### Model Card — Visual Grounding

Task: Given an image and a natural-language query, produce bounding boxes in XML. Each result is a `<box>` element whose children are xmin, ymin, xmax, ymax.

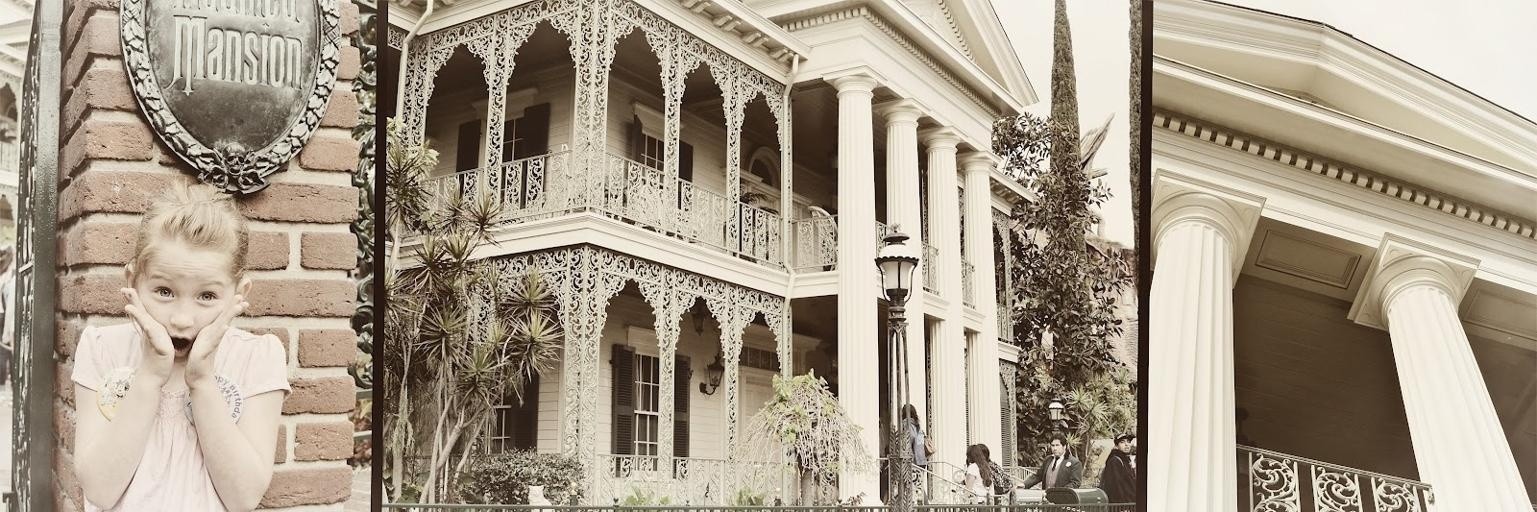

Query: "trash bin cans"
<box><xmin>1011</xmin><ymin>489</ymin><xmax>1110</xmax><ymax>512</ymax></box>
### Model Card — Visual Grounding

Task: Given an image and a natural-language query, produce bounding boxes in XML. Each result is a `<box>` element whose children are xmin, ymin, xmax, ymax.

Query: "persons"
<box><xmin>70</xmin><ymin>179</ymin><xmax>296</xmax><ymax>512</ymax></box>
<box><xmin>1095</xmin><ymin>432</ymin><xmax>1136</xmax><ymax>511</ymax></box>
<box><xmin>1017</xmin><ymin>433</ymin><xmax>1083</xmax><ymax>490</ymax></box>
<box><xmin>887</xmin><ymin>403</ymin><xmax>930</xmax><ymax>504</ymax></box>
<box><xmin>960</xmin><ymin>444</ymin><xmax>996</xmax><ymax>512</ymax></box>
<box><xmin>975</xmin><ymin>442</ymin><xmax>1003</xmax><ymax>511</ymax></box>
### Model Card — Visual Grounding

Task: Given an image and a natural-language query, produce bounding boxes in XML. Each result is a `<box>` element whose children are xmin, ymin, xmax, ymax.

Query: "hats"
<box><xmin>1114</xmin><ymin>433</ymin><xmax>1134</xmax><ymax>446</ymax></box>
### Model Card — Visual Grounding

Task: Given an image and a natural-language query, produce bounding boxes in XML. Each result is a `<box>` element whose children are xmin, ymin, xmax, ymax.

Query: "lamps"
<box><xmin>692</xmin><ymin>303</ymin><xmax>708</xmax><ymax>335</ymax></box>
<box><xmin>699</xmin><ymin>353</ymin><xmax>725</xmax><ymax>396</ymax></box>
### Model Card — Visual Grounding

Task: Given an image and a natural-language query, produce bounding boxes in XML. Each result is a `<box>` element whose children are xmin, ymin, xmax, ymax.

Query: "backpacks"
<box><xmin>987</xmin><ymin>460</ymin><xmax>1015</xmax><ymax>494</ymax></box>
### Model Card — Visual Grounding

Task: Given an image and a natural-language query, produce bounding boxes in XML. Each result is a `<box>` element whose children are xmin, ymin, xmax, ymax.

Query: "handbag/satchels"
<box><xmin>923</xmin><ymin>431</ymin><xmax>937</xmax><ymax>457</ymax></box>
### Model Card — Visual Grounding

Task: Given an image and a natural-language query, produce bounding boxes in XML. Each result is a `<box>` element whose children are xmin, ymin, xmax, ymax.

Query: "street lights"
<box><xmin>1048</xmin><ymin>395</ymin><xmax>1064</xmax><ymax>440</ymax></box>
<box><xmin>873</xmin><ymin>225</ymin><xmax>920</xmax><ymax>512</ymax></box>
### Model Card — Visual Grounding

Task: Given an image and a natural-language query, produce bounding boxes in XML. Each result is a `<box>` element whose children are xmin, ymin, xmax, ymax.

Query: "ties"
<box><xmin>1052</xmin><ymin>456</ymin><xmax>1060</xmax><ymax>472</ymax></box>
